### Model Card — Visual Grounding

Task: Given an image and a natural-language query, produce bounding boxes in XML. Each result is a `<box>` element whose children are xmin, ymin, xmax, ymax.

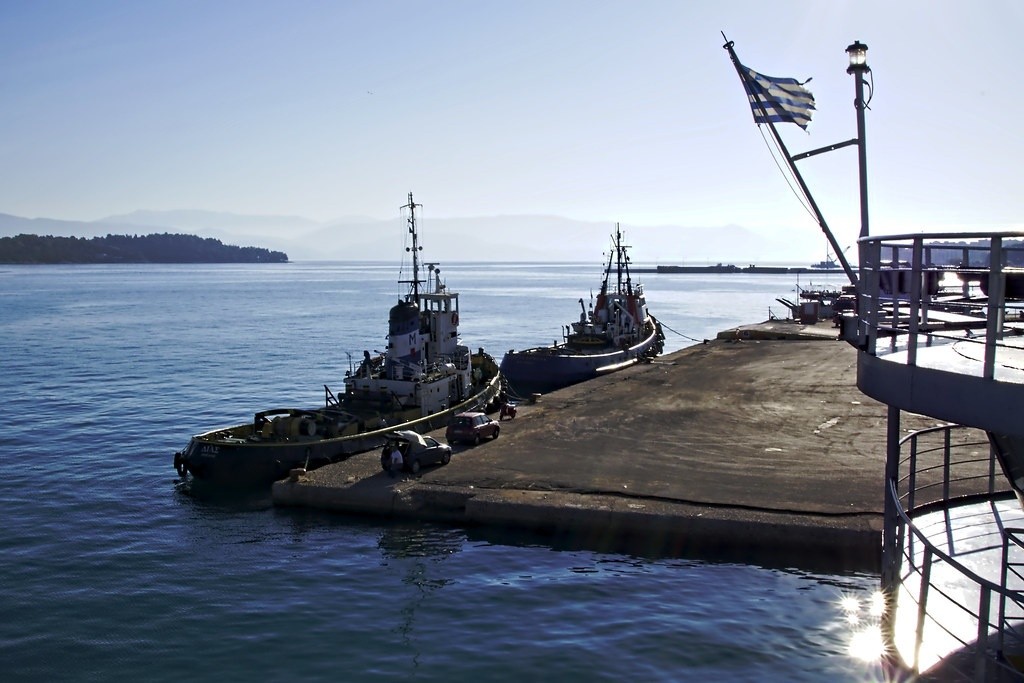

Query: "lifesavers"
<box><xmin>173</xmin><ymin>451</ymin><xmax>188</xmax><ymax>479</ymax></box>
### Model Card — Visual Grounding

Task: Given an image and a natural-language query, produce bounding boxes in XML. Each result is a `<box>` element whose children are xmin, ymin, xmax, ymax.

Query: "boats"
<box><xmin>173</xmin><ymin>188</ymin><xmax>511</xmax><ymax>502</ymax></box>
<box><xmin>499</xmin><ymin>221</ymin><xmax>667</xmax><ymax>404</ymax></box>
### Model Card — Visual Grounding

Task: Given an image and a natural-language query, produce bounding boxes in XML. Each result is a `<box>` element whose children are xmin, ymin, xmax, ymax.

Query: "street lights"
<box><xmin>844</xmin><ymin>38</ymin><xmax>871</xmax><ymax>237</ymax></box>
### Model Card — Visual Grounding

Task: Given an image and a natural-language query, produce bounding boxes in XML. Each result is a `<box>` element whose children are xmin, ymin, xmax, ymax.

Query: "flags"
<box><xmin>730</xmin><ymin>55</ymin><xmax>818</xmax><ymax>136</ymax></box>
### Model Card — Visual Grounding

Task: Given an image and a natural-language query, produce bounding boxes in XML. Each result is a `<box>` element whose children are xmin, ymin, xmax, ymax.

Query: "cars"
<box><xmin>446</xmin><ymin>411</ymin><xmax>501</xmax><ymax>447</ymax></box>
<box><xmin>380</xmin><ymin>430</ymin><xmax>453</xmax><ymax>475</ymax></box>
